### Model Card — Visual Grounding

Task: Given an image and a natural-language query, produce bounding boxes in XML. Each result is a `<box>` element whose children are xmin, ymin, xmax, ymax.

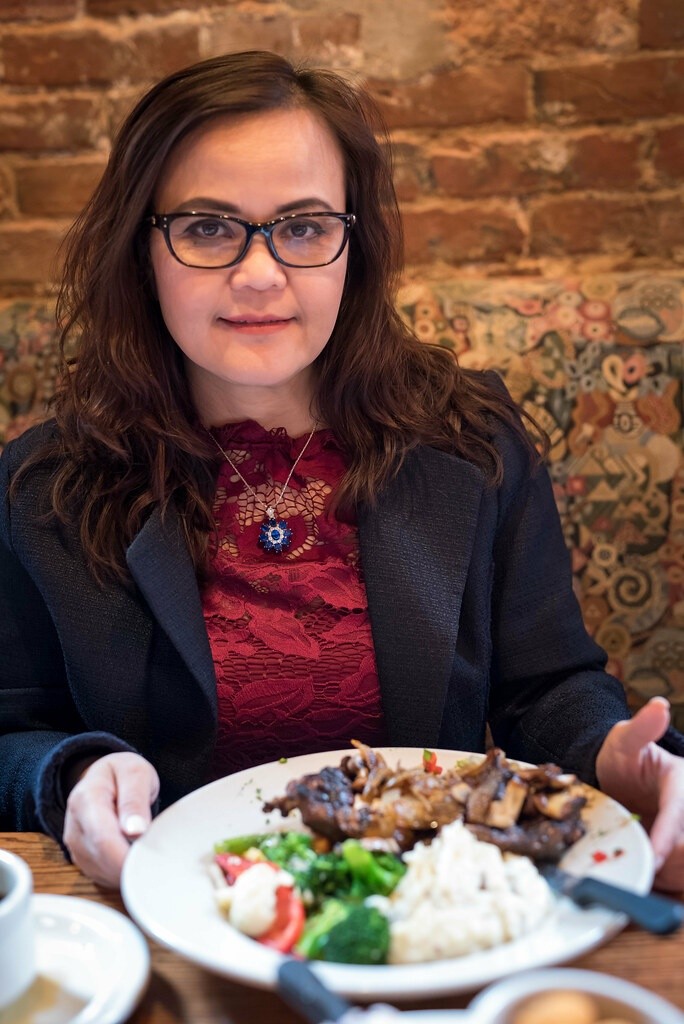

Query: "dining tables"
<box><xmin>0</xmin><ymin>829</ymin><xmax>684</xmax><ymax>1024</ymax></box>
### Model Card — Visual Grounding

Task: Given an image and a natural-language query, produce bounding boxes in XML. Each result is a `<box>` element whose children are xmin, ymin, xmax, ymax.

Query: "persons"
<box><xmin>0</xmin><ymin>48</ymin><xmax>684</xmax><ymax>894</ymax></box>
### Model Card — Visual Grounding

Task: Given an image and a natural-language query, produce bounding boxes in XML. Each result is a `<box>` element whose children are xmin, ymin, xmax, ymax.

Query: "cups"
<box><xmin>368</xmin><ymin>966</ymin><xmax>684</xmax><ymax>1024</ymax></box>
<box><xmin>0</xmin><ymin>847</ymin><xmax>39</xmax><ymax>1002</ymax></box>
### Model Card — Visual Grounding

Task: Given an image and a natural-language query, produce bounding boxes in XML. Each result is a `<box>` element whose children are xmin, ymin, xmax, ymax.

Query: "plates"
<box><xmin>0</xmin><ymin>893</ymin><xmax>150</xmax><ymax>1024</ymax></box>
<box><xmin>121</xmin><ymin>746</ymin><xmax>656</xmax><ymax>999</ymax></box>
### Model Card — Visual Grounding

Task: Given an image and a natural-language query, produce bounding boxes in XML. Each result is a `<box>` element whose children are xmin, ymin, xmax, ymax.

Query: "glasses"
<box><xmin>146</xmin><ymin>212</ymin><xmax>357</xmax><ymax>269</ymax></box>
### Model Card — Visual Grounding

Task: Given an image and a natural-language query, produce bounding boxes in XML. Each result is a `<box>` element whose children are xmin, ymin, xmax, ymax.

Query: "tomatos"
<box><xmin>217</xmin><ymin>849</ymin><xmax>303</xmax><ymax>950</ymax></box>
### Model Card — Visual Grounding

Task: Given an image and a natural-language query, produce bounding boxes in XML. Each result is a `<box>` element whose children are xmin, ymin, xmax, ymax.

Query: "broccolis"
<box><xmin>211</xmin><ymin>829</ymin><xmax>406</xmax><ymax>965</ymax></box>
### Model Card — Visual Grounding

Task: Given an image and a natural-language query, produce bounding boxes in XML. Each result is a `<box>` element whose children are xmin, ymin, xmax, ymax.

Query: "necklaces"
<box><xmin>201</xmin><ymin>415</ymin><xmax>322</xmax><ymax>555</ymax></box>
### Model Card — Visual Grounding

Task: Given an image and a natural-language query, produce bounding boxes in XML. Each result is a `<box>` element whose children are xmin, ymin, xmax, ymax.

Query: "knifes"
<box><xmin>542</xmin><ymin>864</ymin><xmax>684</xmax><ymax>936</ymax></box>
